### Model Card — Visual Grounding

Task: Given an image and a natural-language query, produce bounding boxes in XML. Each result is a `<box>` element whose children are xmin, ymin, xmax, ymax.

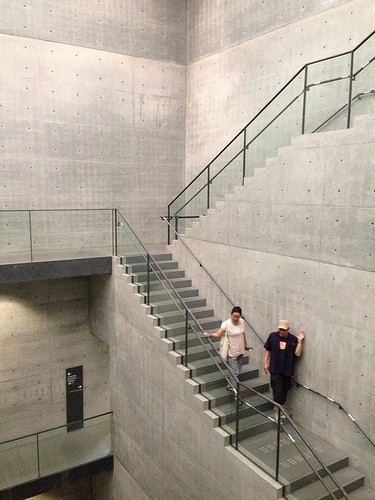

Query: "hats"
<box><xmin>277</xmin><ymin>320</ymin><xmax>290</xmax><ymax>330</ymax></box>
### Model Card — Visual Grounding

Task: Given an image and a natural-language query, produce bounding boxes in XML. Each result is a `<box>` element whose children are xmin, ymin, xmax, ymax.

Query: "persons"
<box><xmin>204</xmin><ymin>307</ymin><xmax>250</xmax><ymax>405</ymax></box>
<box><xmin>263</xmin><ymin>319</ymin><xmax>306</xmax><ymax>415</ymax></box>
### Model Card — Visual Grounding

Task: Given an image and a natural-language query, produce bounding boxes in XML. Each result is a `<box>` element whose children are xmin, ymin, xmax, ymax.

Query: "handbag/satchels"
<box><xmin>219</xmin><ymin>321</ymin><xmax>229</xmax><ymax>363</ymax></box>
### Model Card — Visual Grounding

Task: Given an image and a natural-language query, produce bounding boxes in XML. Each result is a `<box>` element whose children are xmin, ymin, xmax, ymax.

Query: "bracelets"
<box><xmin>298</xmin><ymin>341</ymin><xmax>302</xmax><ymax>343</ymax></box>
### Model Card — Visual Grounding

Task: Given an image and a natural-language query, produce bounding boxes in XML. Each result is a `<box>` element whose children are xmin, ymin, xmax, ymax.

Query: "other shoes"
<box><xmin>226</xmin><ymin>384</ymin><xmax>234</xmax><ymax>392</ymax></box>
<box><xmin>234</xmin><ymin>395</ymin><xmax>244</xmax><ymax>407</ymax></box>
<box><xmin>274</xmin><ymin>407</ymin><xmax>288</xmax><ymax>421</ymax></box>
